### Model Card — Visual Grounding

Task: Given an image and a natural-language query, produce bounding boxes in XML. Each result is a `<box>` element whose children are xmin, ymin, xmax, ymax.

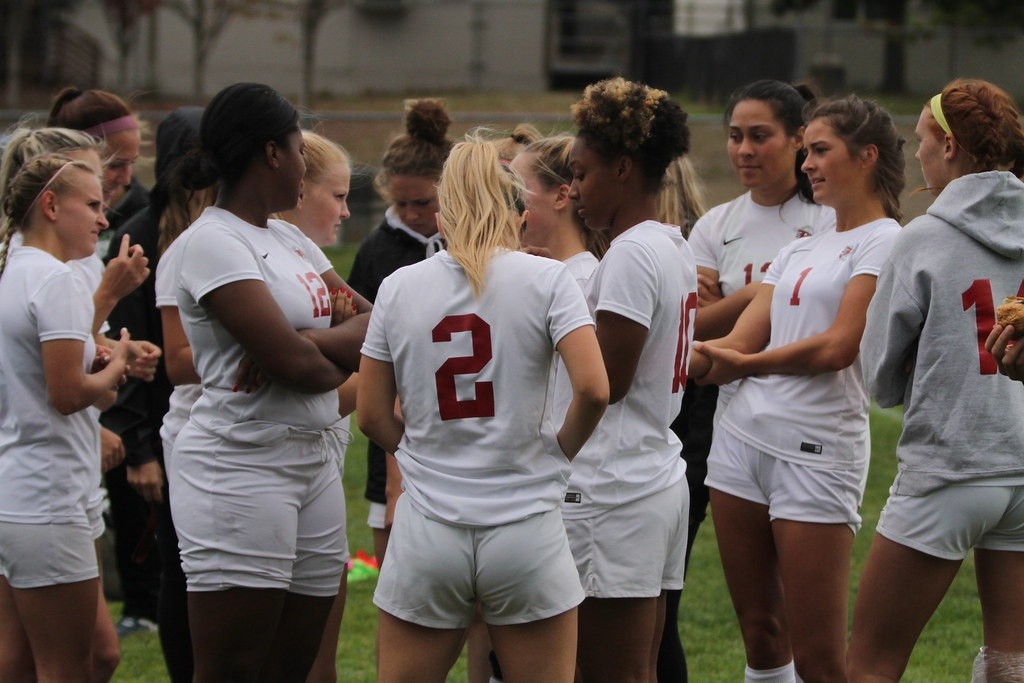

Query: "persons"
<box><xmin>0</xmin><ymin>76</ymin><xmax>1024</xmax><ymax>683</ymax></box>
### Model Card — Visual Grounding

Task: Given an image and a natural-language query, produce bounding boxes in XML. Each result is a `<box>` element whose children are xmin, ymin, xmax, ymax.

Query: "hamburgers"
<box><xmin>995</xmin><ymin>295</ymin><xmax>1024</xmax><ymax>338</ymax></box>
